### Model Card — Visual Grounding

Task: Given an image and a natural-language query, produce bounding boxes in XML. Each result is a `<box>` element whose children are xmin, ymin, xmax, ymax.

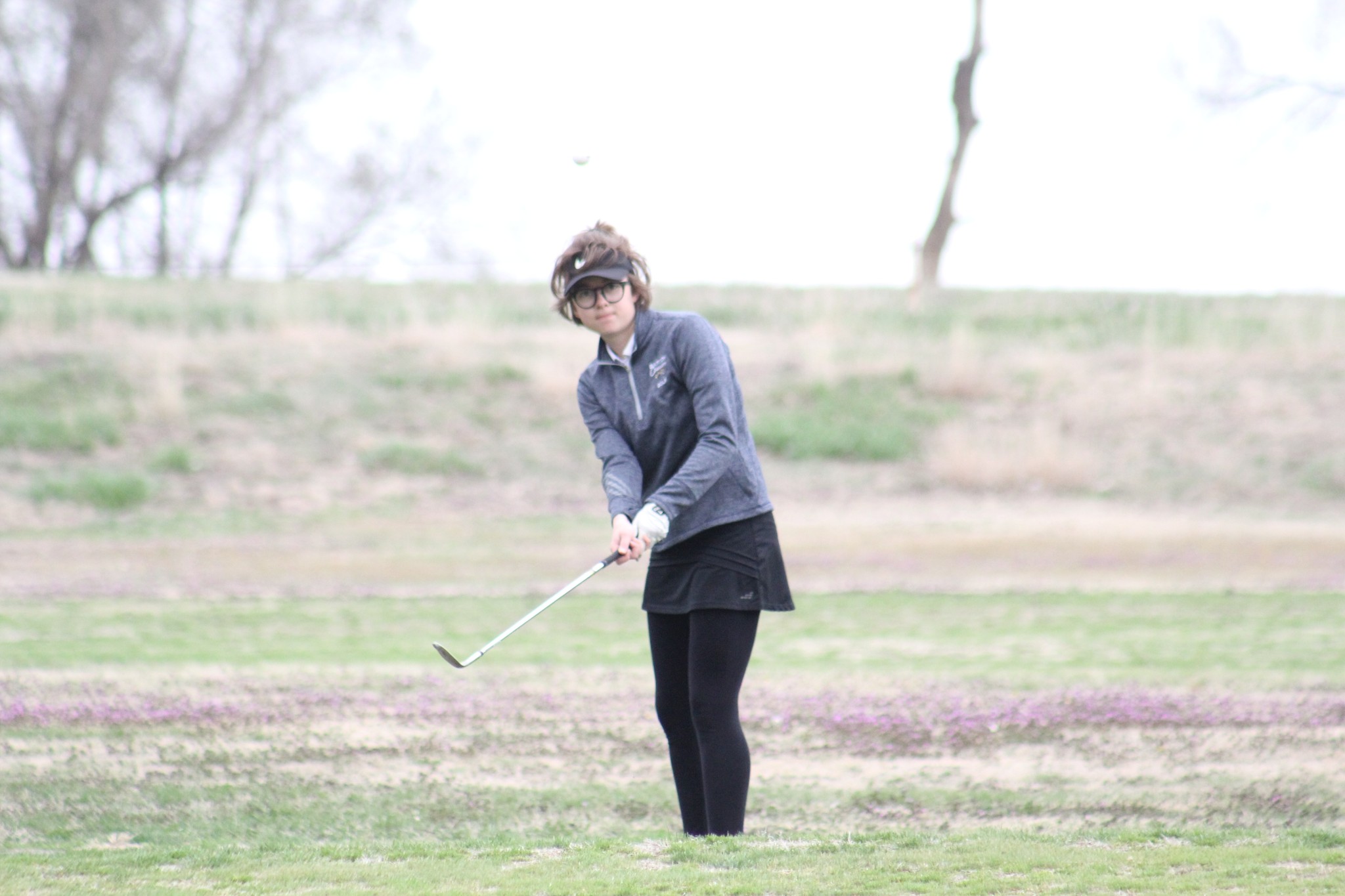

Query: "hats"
<box><xmin>561</xmin><ymin>246</ymin><xmax>633</xmax><ymax>297</ymax></box>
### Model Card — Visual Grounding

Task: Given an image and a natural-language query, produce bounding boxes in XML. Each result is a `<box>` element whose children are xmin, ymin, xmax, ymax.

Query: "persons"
<box><xmin>551</xmin><ymin>224</ymin><xmax>797</xmax><ymax>836</ymax></box>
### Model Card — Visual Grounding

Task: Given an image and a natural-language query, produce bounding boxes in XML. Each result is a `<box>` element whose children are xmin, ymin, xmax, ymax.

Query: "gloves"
<box><xmin>632</xmin><ymin>502</ymin><xmax>670</xmax><ymax>548</ymax></box>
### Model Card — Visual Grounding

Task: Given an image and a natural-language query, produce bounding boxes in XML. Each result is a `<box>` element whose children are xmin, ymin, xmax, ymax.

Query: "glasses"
<box><xmin>573</xmin><ymin>282</ymin><xmax>632</xmax><ymax>309</ymax></box>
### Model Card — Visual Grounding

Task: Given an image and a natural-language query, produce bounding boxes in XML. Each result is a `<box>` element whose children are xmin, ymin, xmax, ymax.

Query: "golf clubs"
<box><xmin>431</xmin><ymin>530</ymin><xmax>648</xmax><ymax>670</ymax></box>
